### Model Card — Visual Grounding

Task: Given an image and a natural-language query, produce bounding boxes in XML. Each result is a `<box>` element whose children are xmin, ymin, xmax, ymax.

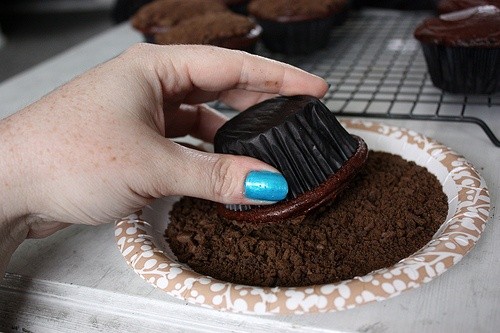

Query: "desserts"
<box><xmin>414</xmin><ymin>4</ymin><xmax>500</xmax><ymax>95</ymax></box>
<box><xmin>130</xmin><ymin>0</ymin><xmax>351</xmax><ymax>54</ymax></box>
<box><xmin>211</xmin><ymin>94</ymin><xmax>369</xmax><ymax>229</ymax></box>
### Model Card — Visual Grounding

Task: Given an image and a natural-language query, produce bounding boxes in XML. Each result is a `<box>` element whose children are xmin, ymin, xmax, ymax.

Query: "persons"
<box><xmin>0</xmin><ymin>41</ymin><xmax>330</xmax><ymax>288</ymax></box>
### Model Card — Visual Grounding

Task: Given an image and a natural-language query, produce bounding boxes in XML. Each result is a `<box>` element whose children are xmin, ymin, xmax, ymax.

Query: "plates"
<box><xmin>111</xmin><ymin>119</ymin><xmax>489</xmax><ymax>318</ymax></box>
